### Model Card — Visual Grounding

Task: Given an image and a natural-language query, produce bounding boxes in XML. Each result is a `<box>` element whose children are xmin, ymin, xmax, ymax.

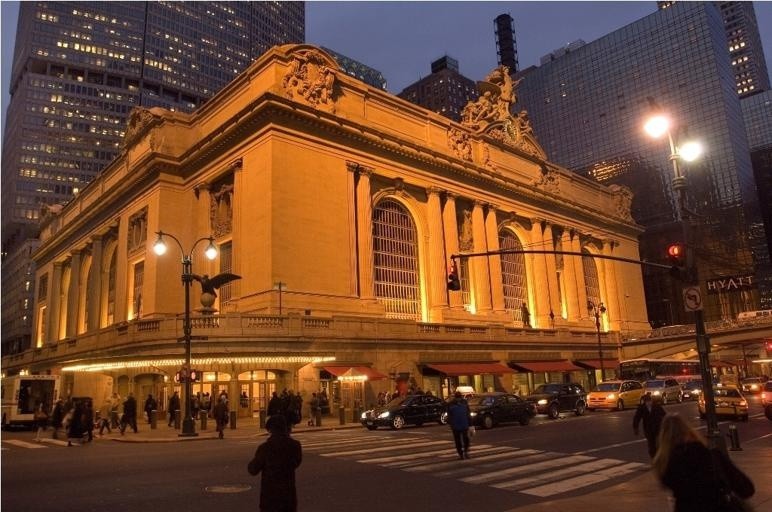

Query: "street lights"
<box><xmin>587</xmin><ymin>299</ymin><xmax>606</xmax><ymax>383</ymax></box>
<box><xmin>642</xmin><ymin>93</ymin><xmax>728</xmax><ymax>458</ymax></box>
<box><xmin>153</xmin><ymin>229</ymin><xmax>227</xmax><ymax>440</ymax></box>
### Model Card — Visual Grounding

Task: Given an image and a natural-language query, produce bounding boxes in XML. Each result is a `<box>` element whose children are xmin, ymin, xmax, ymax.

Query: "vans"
<box><xmin>737</xmin><ymin>309</ymin><xmax>772</xmax><ymax>327</ymax></box>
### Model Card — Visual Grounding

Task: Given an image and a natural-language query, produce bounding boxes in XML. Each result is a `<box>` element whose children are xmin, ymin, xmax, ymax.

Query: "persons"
<box><xmin>520</xmin><ymin>302</ymin><xmax>530</xmax><ymax>325</ymax></box>
<box><xmin>32</xmin><ymin>387</ymin><xmax>328</xmax><ymax>447</ymax></box>
<box><xmin>371</xmin><ymin>385</ymin><xmax>432</xmax><ymax>410</ymax></box>
<box><xmin>447</xmin><ymin>391</ymin><xmax>474</xmax><ymax>460</ymax></box>
<box><xmin>247</xmin><ymin>415</ymin><xmax>302</xmax><ymax>512</ymax></box>
<box><xmin>652</xmin><ymin>412</ymin><xmax>755</xmax><ymax>512</ymax></box>
<box><xmin>463</xmin><ymin>64</ymin><xmax>534</xmax><ymax>136</ymax></box>
<box><xmin>632</xmin><ymin>395</ymin><xmax>667</xmax><ymax>459</ymax></box>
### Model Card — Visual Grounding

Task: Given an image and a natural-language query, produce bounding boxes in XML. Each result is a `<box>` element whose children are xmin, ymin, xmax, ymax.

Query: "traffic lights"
<box><xmin>190</xmin><ymin>369</ymin><xmax>197</xmax><ymax>382</ymax></box>
<box><xmin>444</xmin><ymin>264</ymin><xmax>461</xmax><ymax>292</ymax></box>
<box><xmin>175</xmin><ymin>371</ymin><xmax>182</xmax><ymax>382</ymax></box>
<box><xmin>663</xmin><ymin>225</ymin><xmax>692</xmax><ymax>282</ymax></box>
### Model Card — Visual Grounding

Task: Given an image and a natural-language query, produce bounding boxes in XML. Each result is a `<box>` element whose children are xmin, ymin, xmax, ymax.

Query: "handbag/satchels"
<box><xmin>723</xmin><ymin>490</ymin><xmax>754</xmax><ymax>512</ymax></box>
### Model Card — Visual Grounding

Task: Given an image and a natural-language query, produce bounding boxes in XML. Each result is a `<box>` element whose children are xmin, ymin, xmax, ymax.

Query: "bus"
<box><xmin>616</xmin><ymin>356</ymin><xmax>710</xmax><ymax>387</ymax></box>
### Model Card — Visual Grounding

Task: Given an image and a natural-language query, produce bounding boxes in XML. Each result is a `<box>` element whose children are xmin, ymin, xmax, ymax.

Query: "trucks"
<box><xmin>1</xmin><ymin>374</ymin><xmax>64</xmax><ymax>429</ymax></box>
